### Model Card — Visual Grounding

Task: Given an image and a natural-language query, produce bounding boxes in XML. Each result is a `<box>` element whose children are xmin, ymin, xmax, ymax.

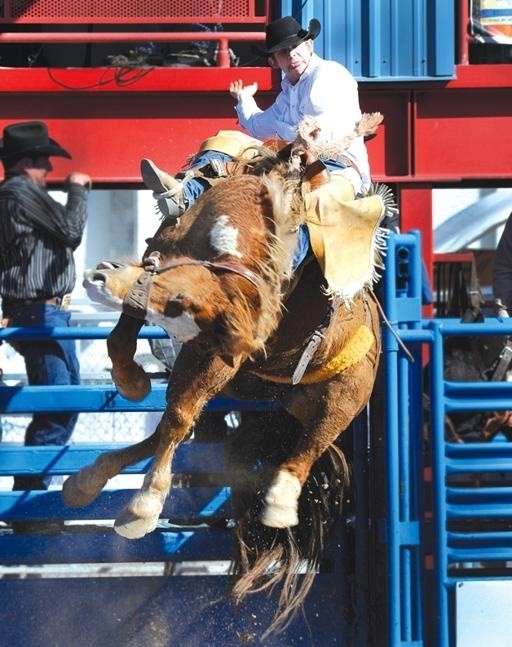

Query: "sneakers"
<box><xmin>11</xmin><ymin>520</ymin><xmax>60</xmax><ymax>535</ymax></box>
<box><xmin>139</xmin><ymin>158</ymin><xmax>186</xmax><ymax>218</ymax></box>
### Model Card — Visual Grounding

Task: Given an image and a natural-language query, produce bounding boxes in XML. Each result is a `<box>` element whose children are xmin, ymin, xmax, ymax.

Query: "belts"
<box><xmin>10</xmin><ymin>295</ymin><xmax>57</xmax><ymax>306</ymax></box>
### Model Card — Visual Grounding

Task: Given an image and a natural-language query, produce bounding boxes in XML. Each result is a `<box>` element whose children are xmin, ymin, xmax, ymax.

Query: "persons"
<box><xmin>0</xmin><ymin>122</ymin><xmax>92</xmax><ymax>534</ymax></box>
<box><xmin>141</xmin><ymin>16</ymin><xmax>371</xmax><ymax>272</ymax></box>
<box><xmin>493</xmin><ymin>211</ymin><xmax>512</xmax><ymax>317</ymax></box>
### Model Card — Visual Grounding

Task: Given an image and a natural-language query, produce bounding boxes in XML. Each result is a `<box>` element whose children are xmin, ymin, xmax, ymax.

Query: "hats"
<box><xmin>263</xmin><ymin>15</ymin><xmax>321</xmax><ymax>53</ymax></box>
<box><xmin>0</xmin><ymin>120</ymin><xmax>73</xmax><ymax>162</ymax></box>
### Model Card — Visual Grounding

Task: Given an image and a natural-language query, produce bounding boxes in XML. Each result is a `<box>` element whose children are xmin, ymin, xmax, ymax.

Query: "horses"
<box><xmin>57</xmin><ymin>164</ymin><xmax>387</xmax><ymax>643</ymax></box>
